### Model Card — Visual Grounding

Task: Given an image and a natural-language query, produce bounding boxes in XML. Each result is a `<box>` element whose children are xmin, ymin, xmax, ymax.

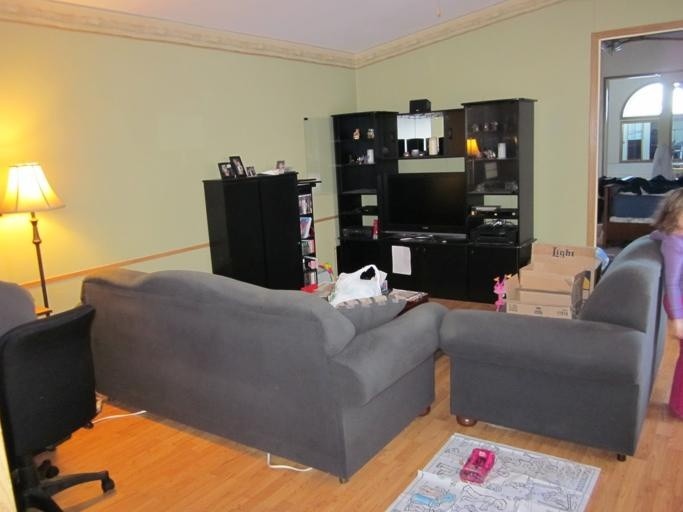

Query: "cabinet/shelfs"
<box><xmin>460</xmin><ymin>98</ymin><xmax>537</xmax><ymax>246</ymax></box>
<box><xmin>330</xmin><ymin>111</ymin><xmax>399</xmax><ymax>245</ymax></box>
<box><xmin>336</xmin><ymin>234</ymin><xmax>519</xmax><ymax>304</ymax></box>
<box><xmin>297</xmin><ymin>184</ymin><xmax>319</xmax><ymax>286</ymax></box>
<box><xmin>203</xmin><ymin>171</ymin><xmax>304</xmax><ymax>290</ymax></box>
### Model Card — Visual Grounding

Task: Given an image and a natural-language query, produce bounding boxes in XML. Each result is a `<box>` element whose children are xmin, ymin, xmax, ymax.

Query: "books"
<box><xmin>301</xmin><ymin>217</ymin><xmax>313</xmax><ymax>238</ymax></box>
<box><xmin>299</xmin><ymin>193</ymin><xmax>314</xmax><ymax>215</ymax></box>
<box><xmin>304</xmin><ymin>271</ymin><xmax>318</xmax><ymax>287</ymax></box>
<box><xmin>302</xmin><ymin>239</ymin><xmax>315</xmax><ymax>255</ymax></box>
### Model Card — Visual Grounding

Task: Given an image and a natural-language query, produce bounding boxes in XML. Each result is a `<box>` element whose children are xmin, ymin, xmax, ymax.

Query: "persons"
<box><xmin>649</xmin><ymin>188</ymin><xmax>683</xmax><ymax>418</ymax></box>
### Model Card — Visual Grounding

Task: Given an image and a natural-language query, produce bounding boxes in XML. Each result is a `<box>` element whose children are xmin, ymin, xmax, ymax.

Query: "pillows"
<box><xmin>335</xmin><ymin>296</ymin><xmax>407</xmax><ymax>332</ymax></box>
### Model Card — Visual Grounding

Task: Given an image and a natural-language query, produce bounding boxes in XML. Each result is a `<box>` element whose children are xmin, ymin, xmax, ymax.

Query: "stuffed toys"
<box><xmin>493</xmin><ymin>274</ymin><xmax>512</xmax><ymax>312</ymax></box>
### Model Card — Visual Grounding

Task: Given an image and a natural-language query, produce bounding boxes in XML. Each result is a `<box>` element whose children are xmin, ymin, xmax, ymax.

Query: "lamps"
<box><xmin>0</xmin><ymin>161</ymin><xmax>65</xmax><ymax>316</ymax></box>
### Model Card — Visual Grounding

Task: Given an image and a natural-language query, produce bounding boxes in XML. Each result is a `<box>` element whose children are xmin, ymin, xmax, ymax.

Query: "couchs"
<box><xmin>441</xmin><ymin>234</ymin><xmax>667</xmax><ymax>461</ymax></box>
<box><xmin>79</xmin><ymin>268</ymin><xmax>448</xmax><ymax>483</ymax></box>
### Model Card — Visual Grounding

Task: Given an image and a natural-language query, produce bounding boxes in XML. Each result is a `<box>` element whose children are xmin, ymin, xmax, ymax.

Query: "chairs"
<box><xmin>0</xmin><ymin>305</ymin><xmax>116</xmax><ymax>512</ymax></box>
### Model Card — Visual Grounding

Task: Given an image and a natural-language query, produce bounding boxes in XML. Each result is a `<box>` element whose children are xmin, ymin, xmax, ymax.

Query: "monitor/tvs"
<box><xmin>383</xmin><ymin>172</ymin><xmax>468</xmax><ymax>240</ymax></box>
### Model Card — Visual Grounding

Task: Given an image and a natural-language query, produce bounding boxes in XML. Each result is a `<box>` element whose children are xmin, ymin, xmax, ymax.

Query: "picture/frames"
<box><xmin>230</xmin><ymin>156</ymin><xmax>246</xmax><ymax>177</ymax></box>
<box><xmin>218</xmin><ymin>162</ymin><xmax>237</xmax><ymax>179</ymax></box>
